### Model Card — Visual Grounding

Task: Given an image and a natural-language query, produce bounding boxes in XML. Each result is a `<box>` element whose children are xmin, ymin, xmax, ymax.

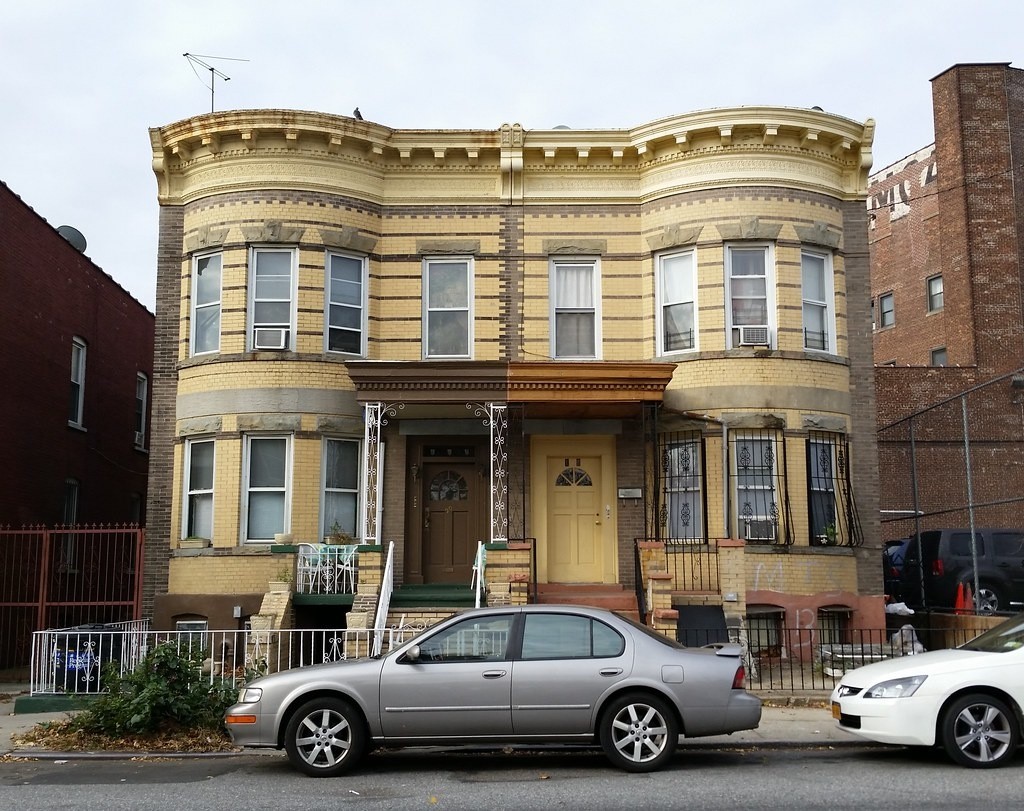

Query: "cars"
<box><xmin>224</xmin><ymin>603</ymin><xmax>762</xmax><ymax>778</ymax></box>
<box><xmin>830</xmin><ymin>613</ymin><xmax>1024</xmax><ymax>768</ymax></box>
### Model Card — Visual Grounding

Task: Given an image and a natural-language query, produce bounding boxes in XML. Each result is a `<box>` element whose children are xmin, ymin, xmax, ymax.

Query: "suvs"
<box><xmin>881</xmin><ymin>528</ymin><xmax>1024</xmax><ymax>615</ymax></box>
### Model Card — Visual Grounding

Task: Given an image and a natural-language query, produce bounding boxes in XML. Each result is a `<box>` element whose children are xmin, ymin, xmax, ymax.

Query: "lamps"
<box><xmin>410</xmin><ymin>463</ymin><xmax>420</xmax><ymax>479</ymax></box>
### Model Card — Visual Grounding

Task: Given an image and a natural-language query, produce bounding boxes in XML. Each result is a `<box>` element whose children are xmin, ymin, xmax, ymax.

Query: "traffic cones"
<box><xmin>954</xmin><ymin>582</ymin><xmax>965</xmax><ymax>614</ymax></box>
<box><xmin>964</xmin><ymin>583</ymin><xmax>975</xmax><ymax>614</ymax></box>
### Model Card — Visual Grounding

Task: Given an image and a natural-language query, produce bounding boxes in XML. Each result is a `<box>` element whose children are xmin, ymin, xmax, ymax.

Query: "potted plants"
<box><xmin>250</xmin><ymin>611</ymin><xmax>278</xmax><ymax>636</ymax></box>
<box><xmin>346</xmin><ymin>608</ymin><xmax>369</xmax><ymax>629</ymax></box>
<box><xmin>324</xmin><ymin>519</ymin><xmax>360</xmax><ymax>545</ymax></box>
<box><xmin>357</xmin><ymin>579</ymin><xmax>380</xmax><ymax>594</ymax></box>
<box><xmin>268</xmin><ymin>568</ymin><xmax>294</xmax><ymax>591</ymax></box>
<box><xmin>179</xmin><ymin>536</ymin><xmax>210</xmax><ymax>549</ymax></box>
<box><xmin>815</xmin><ymin>523</ymin><xmax>838</xmax><ymax>546</ymax></box>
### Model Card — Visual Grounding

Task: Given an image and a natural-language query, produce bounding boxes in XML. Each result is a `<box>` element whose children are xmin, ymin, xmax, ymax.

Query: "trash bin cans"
<box><xmin>52</xmin><ymin>623</ymin><xmax>124</xmax><ymax>692</ymax></box>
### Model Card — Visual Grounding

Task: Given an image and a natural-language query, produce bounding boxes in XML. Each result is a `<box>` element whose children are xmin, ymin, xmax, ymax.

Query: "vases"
<box><xmin>274</xmin><ymin>533</ymin><xmax>294</xmax><ymax>544</ymax></box>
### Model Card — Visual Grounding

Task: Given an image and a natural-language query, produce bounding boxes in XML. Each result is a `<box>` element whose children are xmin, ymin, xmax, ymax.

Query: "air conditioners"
<box><xmin>745</xmin><ymin>518</ymin><xmax>776</xmax><ymax>540</ymax></box>
<box><xmin>738</xmin><ymin>325</ymin><xmax>771</xmax><ymax>346</ymax></box>
<box><xmin>255</xmin><ymin>328</ymin><xmax>286</xmax><ymax>349</ymax></box>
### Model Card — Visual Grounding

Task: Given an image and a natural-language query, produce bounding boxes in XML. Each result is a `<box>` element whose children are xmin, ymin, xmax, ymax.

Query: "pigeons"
<box><xmin>352</xmin><ymin>106</ymin><xmax>363</xmax><ymax>120</ymax></box>
